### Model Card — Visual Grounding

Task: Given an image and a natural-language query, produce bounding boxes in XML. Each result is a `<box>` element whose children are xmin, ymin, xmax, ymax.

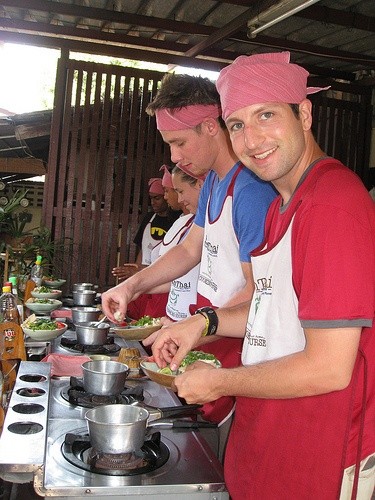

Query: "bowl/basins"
<box><xmin>22</xmin><ymin>323</ymin><xmax>68</xmax><ymax>341</ymax></box>
<box><xmin>114</xmin><ymin>325</ymin><xmax>163</xmax><ymax>341</ymax></box>
<box><xmin>140</xmin><ymin>351</ymin><xmax>221</xmax><ymax>388</ymax></box>
<box><xmin>89</xmin><ymin>355</ymin><xmax>111</xmax><ymax>361</ymax></box>
<box><xmin>31</xmin><ymin>290</ymin><xmax>62</xmax><ymax>299</ymax></box>
<box><xmin>25</xmin><ymin>298</ymin><xmax>62</xmax><ymax>314</ymax></box>
<box><xmin>44</xmin><ymin>279</ymin><xmax>66</xmax><ymax>288</ymax></box>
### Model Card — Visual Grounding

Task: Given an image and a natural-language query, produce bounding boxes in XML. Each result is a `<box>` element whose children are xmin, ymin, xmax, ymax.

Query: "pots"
<box><xmin>73</xmin><ymin>291</ymin><xmax>97</xmax><ymax>306</ymax></box>
<box><xmin>85</xmin><ymin>404</ymin><xmax>203</xmax><ymax>454</ymax></box>
<box><xmin>72</xmin><ymin>307</ymin><xmax>102</xmax><ymax>323</ymax></box>
<box><xmin>75</xmin><ymin>322</ymin><xmax>113</xmax><ymax>345</ymax></box>
<box><xmin>81</xmin><ymin>360</ymin><xmax>142</xmax><ymax>397</ymax></box>
<box><xmin>73</xmin><ymin>283</ymin><xmax>94</xmax><ymax>291</ymax></box>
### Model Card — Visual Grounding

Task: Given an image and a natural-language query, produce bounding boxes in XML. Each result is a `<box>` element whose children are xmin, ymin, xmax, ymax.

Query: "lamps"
<box><xmin>247</xmin><ymin>0</ymin><xmax>320</xmax><ymax>39</ymax></box>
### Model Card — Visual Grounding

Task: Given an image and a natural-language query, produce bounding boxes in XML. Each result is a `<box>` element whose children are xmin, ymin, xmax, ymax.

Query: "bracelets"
<box><xmin>194</xmin><ymin>306</ymin><xmax>219</xmax><ymax>336</ymax></box>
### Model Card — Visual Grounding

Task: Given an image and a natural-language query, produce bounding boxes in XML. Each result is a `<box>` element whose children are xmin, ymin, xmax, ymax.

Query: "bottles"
<box><xmin>0</xmin><ymin>277</ymin><xmax>24</xmax><ymax>341</ymax></box>
<box><xmin>30</xmin><ymin>256</ymin><xmax>43</xmax><ymax>288</ymax></box>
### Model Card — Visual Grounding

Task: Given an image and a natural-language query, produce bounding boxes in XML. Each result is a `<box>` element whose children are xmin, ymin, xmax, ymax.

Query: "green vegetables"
<box><xmin>129</xmin><ymin>315</ymin><xmax>154</xmax><ymax>326</ymax></box>
<box><xmin>39</xmin><ymin>286</ymin><xmax>52</xmax><ymax>294</ymax></box>
<box><xmin>28</xmin><ymin>318</ymin><xmax>59</xmax><ymax>332</ymax></box>
<box><xmin>50</xmin><ymin>274</ymin><xmax>59</xmax><ymax>281</ymax></box>
<box><xmin>33</xmin><ymin>298</ymin><xmax>53</xmax><ymax>304</ymax></box>
<box><xmin>168</xmin><ymin>351</ymin><xmax>221</xmax><ymax>374</ymax></box>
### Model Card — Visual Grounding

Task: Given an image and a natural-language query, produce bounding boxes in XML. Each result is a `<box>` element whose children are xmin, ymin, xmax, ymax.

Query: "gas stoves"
<box><xmin>44</xmin><ymin>287</ymin><xmax>228</xmax><ymax>494</ymax></box>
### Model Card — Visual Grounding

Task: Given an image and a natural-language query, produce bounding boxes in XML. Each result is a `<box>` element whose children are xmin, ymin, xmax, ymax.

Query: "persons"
<box><xmin>113</xmin><ymin>163</ymin><xmax>209</xmax><ymax>325</ymax></box>
<box><xmin>151</xmin><ymin>50</ymin><xmax>375</xmax><ymax>500</ymax></box>
<box><xmin>101</xmin><ymin>71</ymin><xmax>282</xmax><ymax>427</ymax></box>
